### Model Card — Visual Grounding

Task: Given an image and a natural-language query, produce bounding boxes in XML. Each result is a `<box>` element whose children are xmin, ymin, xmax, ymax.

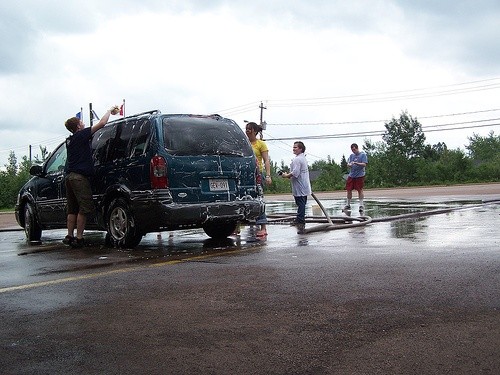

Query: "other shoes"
<box><xmin>70</xmin><ymin>238</ymin><xmax>88</xmax><ymax>248</ymax></box>
<box><xmin>359</xmin><ymin>206</ymin><xmax>364</xmax><ymax>211</ymax></box>
<box><xmin>257</xmin><ymin>229</ymin><xmax>267</xmax><ymax>236</ymax></box>
<box><xmin>345</xmin><ymin>205</ymin><xmax>350</xmax><ymax>209</ymax></box>
<box><xmin>291</xmin><ymin>219</ymin><xmax>305</xmax><ymax>225</ymax></box>
<box><xmin>62</xmin><ymin>235</ymin><xmax>72</xmax><ymax>244</ymax></box>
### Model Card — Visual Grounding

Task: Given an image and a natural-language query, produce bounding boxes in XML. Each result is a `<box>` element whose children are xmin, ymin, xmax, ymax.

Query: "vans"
<box><xmin>14</xmin><ymin>108</ymin><xmax>269</xmax><ymax>250</ymax></box>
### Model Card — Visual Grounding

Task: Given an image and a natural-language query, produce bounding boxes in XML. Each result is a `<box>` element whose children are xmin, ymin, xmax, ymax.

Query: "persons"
<box><xmin>343</xmin><ymin>143</ymin><xmax>368</xmax><ymax>211</ymax></box>
<box><xmin>63</xmin><ymin>105</ymin><xmax>120</xmax><ymax>248</ymax></box>
<box><xmin>281</xmin><ymin>141</ymin><xmax>311</xmax><ymax>225</ymax></box>
<box><xmin>245</xmin><ymin>122</ymin><xmax>272</xmax><ymax>236</ymax></box>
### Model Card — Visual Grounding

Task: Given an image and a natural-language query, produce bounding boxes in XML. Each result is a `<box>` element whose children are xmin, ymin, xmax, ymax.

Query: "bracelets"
<box><xmin>265</xmin><ymin>174</ymin><xmax>270</xmax><ymax>178</ymax></box>
